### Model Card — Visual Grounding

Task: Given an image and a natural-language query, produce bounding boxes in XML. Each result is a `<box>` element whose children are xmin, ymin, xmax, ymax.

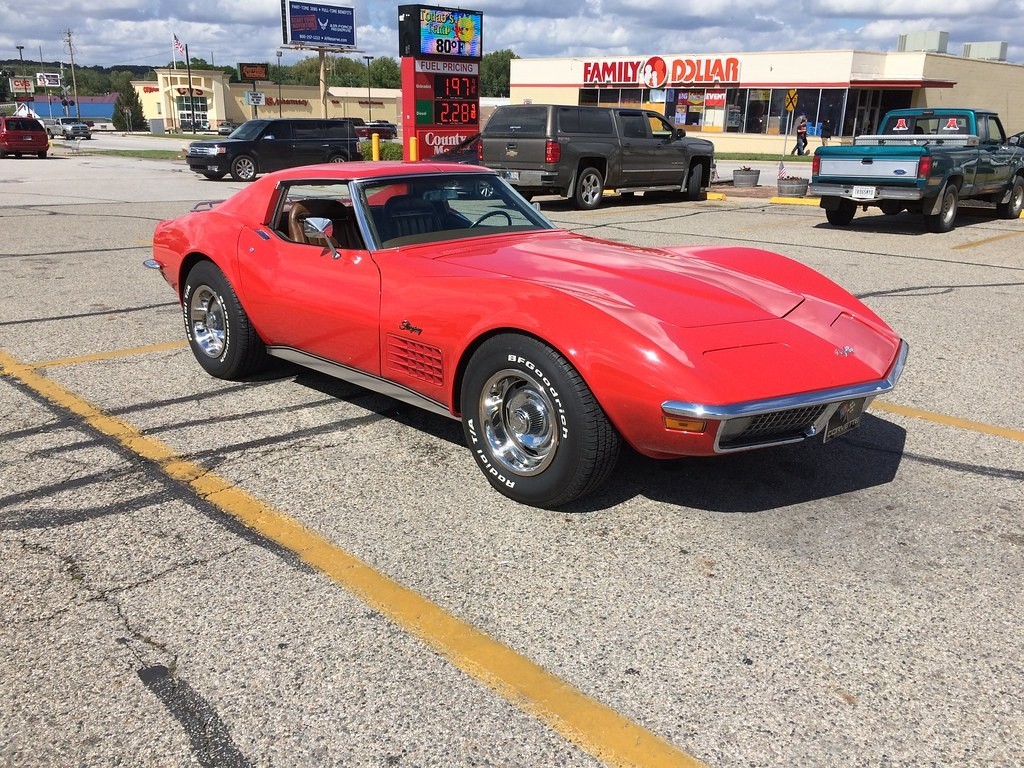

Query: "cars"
<box><xmin>429</xmin><ymin>132</ymin><xmax>560</xmax><ymax>211</ymax></box>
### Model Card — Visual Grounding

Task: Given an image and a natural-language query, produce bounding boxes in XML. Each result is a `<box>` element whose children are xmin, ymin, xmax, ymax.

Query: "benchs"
<box><xmin>279</xmin><ymin>206</ymin><xmax>381</xmax><ymax>234</ymax></box>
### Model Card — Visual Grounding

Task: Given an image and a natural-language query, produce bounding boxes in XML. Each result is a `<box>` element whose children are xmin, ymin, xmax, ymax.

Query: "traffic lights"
<box><xmin>62</xmin><ymin>99</ymin><xmax>68</xmax><ymax>106</ymax></box>
<box><xmin>68</xmin><ymin>100</ymin><xmax>74</xmax><ymax>106</ymax></box>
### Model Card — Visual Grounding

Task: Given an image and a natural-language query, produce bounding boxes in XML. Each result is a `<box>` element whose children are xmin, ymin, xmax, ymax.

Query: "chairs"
<box><xmin>289</xmin><ymin>200</ymin><xmax>363</xmax><ymax>249</ymax></box>
<box><xmin>382</xmin><ymin>194</ymin><xmax>444</xmax><ymax>241</ymax></box>
<box><xmin>906</xmin><ymin>126</ymin><xmax>924</xmax><ymax>134</ymax></box>
<box><xmin>623</xmin><ymin>122</ymin><xmax>645</xmax><ymax>137</ymax></box>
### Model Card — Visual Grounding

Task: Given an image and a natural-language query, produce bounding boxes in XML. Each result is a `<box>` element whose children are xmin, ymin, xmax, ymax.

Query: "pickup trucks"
<box><xmin>809</xmin><ymin>107</ymin><xmax>1024</xmax><ymax>234</ymax></box>
<box><xmin>329</xmin><ymin>115</ymin><xmax>392</xmax><ymax>142</ymax></box>
<box><xmin>217</xmin><ymin>121</ymin><xmax>233</xmax><ymax>135</ymax></box>
<box><xmin>45</xmin><ymin>116</ymin><xmax>92</xmax><ymax>140</ymax></box>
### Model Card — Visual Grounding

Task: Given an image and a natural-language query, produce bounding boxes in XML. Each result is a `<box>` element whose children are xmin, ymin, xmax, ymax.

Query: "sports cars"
<box><xmin>140</xmin><ymin>159</ymin><xmax>912</xmax><ymax>512</ymax></box>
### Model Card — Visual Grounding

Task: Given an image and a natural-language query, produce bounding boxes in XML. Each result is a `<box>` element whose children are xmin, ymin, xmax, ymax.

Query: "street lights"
<box><xmin>15</xmin><ymin>44</ymin><xmax>30</xmax><ymax>114</ymax></box>
<box><xmin>362</xmin><ymin>54</ymin><xmax>376</xmax><ymax>123</ymax></box>
<box><xmin>276</xmin><ymin>51</ymin><xmax>283</xmax><ymax>118</ymax></box>
<box><xmin>168</xmin><ymin>65</ymin><xmax>177</xmax><ymax>133</ymax></box>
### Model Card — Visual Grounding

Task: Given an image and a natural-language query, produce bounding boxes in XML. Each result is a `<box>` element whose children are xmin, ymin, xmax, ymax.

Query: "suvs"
<box><xmin>364</xmin><ymin>119</ymin><xmax>398</xmax><ymax>139</ymax></box>
<box><xmin>0</xmin><ymin>110</ymin><xmax>50</xmax><ymax>160</ymax></box>
<box><xmin>477</xmin><ymin>103</ymin><xmax>717</xmax><ymax>211</ymax></box>
<box><xmin>185</xmin><ymin>117</ymin><xmax>364</xmax><ymax>183</ymax></box>
